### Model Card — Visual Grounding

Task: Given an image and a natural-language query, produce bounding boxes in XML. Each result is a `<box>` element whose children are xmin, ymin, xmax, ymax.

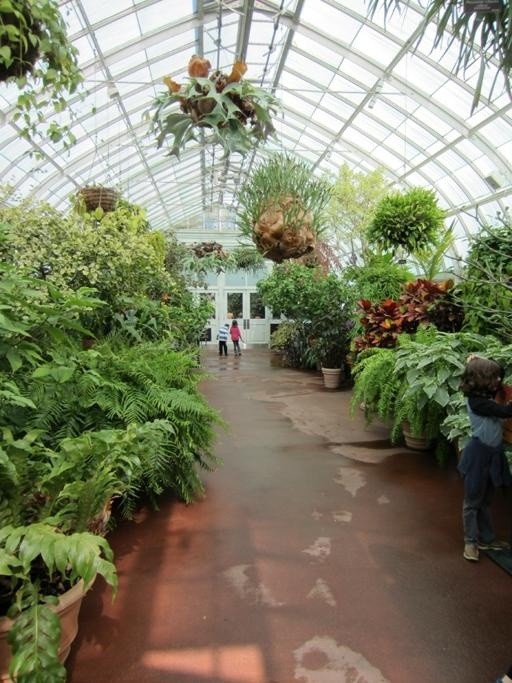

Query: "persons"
<box><xmin>457</xmin><ymin>354</ymin><xmax>512</xmax><ymax>561</ymax></box>
<box><xmin>216</xmin><ymin>324</ymin><xmax>230</xmax><ymax>356</ymax></box>
<box><xmin>229</xmin><ymin>320</ymin><xmax>245</xmax><ymax>356</ymax></box>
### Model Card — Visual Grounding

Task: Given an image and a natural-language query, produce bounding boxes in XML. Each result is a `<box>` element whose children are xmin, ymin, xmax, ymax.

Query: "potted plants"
<box><xmin>0</xmin><ymin>204</ymin><xmax>219</xmax><ymax>539</ymax></box>
<box><xmin>0</xmin><ymin>520</ymin><xmax>117</xmax><ymax>682</ymax></box>
<box><xmin>312</xmin><ymin>311</ymin><xmax>346</xmax><ymax>389</ymax></box>
<box><xmin>140</xmin><ymin>54</ymin><xmax>286</xmax><ymax>165</ymax></box>
<box><xmin>2</xmin><ymin>2</ymin><xmax>87</xmax><ymax>166</ymax></box>
<box><xmin>77</xmin><ymin>187</ymin><xmax>114</xmax><ymax>211</ymax></box>
<box><xmin>237</xmin><ymin>154</ymin><xmax>335</xmax><ymax>260</ymax></box>
<box><xmin>348</xmin><ymin>160</ymin><xmax>509</xmax><ymax>469</ymax></box>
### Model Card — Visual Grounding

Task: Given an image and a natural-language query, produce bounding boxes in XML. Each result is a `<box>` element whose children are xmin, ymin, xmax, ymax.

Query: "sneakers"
<box><xmin>463</xmin><ymin>539</ymin><xmax>508</xmax><ymax>561</ymax></box>
